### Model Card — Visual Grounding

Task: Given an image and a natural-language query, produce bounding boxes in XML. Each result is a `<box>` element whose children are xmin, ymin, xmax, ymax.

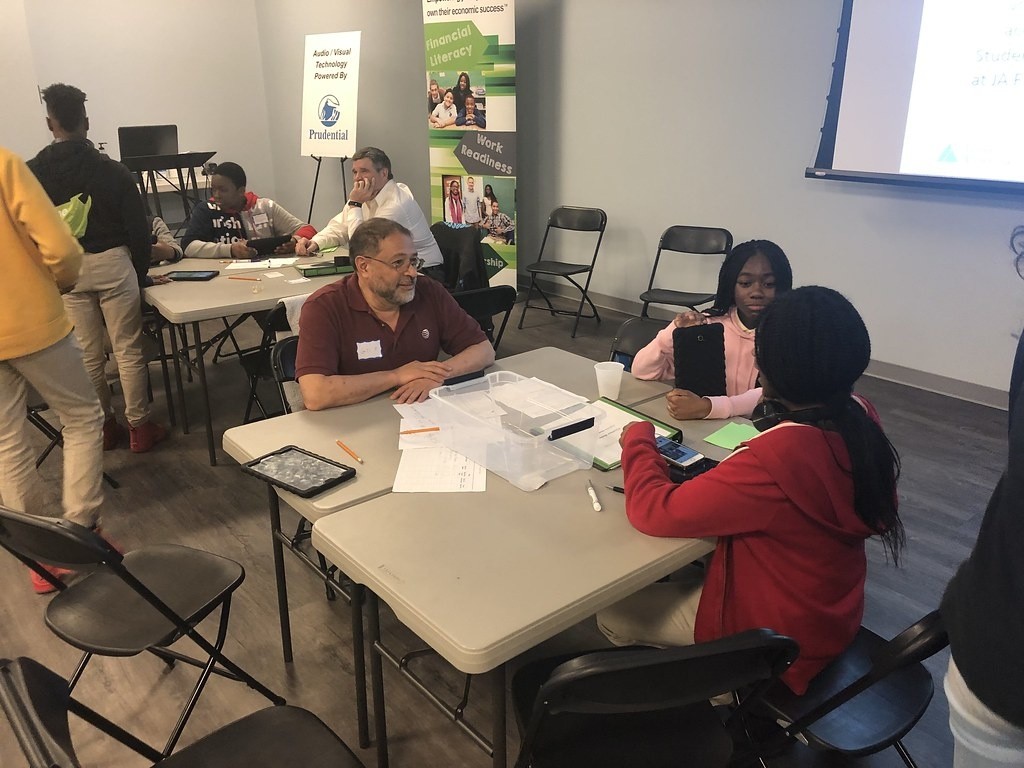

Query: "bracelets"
<box><xmin>348</xmin><ymin>201</ymin><xmax>361</xmax><ymax>207</ymax></box>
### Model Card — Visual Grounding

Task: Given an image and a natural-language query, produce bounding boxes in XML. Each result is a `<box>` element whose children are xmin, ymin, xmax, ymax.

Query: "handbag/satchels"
<box><xmin>55</xmin><ymin>148</ymin><xmax>102</xmax><ymax>239</ymax></box>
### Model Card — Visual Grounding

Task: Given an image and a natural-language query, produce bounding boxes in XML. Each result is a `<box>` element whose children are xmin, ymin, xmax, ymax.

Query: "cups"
<box><xmin>594</xmin><ymin>361</ymin><xmax>625</xmax><ymax>400</ymax></box>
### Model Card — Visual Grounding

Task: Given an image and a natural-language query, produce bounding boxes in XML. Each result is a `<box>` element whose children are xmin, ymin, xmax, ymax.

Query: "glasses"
<box><xmin>364</xmin><ymin>256</ymin><xmax>425</xmax><ymax>273</ymax></box>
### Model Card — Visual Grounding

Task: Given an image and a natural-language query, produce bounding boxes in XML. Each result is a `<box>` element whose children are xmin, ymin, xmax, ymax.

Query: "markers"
<box><xmin>585</xmin><ymin>479</ymin><xmax>601</xmax><ymax>512</ymax></box>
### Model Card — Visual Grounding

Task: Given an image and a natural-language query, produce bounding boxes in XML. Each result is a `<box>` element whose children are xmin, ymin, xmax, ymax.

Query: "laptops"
<box><xmin>118</xmin><ymin>124</ymin><xmax>178</xmax><ymax>159</ymax></box>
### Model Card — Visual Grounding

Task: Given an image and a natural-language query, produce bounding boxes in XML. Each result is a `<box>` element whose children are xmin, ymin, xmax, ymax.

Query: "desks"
<box><xmin>220</xmin><ymin>345</ymin><xmax>671</xmax><ymax>745</ymax></box>
<box><xmin>143</xmin><ymin>246</ymin><xmax>421</xmax><ymax>468</ymax></box>
<box><xmin>123</xmin><ymin>174</ymin><xmax>230</xmax><ymax>193</ymax></box>
<box><xmin>299</xmin><ymin>390</ymin><xmax>775</xmax><ymax>767</ymax></box>
<box><xmin>137</xmin><ymin>247</ymin><xmax>351</xmax><ymax>428</ymax></box>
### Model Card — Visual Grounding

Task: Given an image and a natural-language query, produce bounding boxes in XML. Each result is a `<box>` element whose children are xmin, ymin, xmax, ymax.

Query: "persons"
<box><xmin>430</xmin><ymin>88</ymin><xmax>458</xmax><ymax>128</ymax></box>
<box><xmin>938</xmin><ymin>225</ymin><xmax>1024</xmax><ymax>768</ymax></box>
<box><xmin>631</xmin><ymin>239</ymin><xmax>793</xmax><ymax>420</ymax></box>
<box><xmin>452</xmin><ymin>72</ymin><xmax>473</xmax><ymax>114</ymax></box>
<box><xmin>595</xmin><ymin>285</ymin><xmax>905</xmax><ymax>699</ymax></box>
<box><xmin>461</xmin><ymin>177</ymin><xmax>481</xmax><ymax>228</ymax></box>
<box><xmin>180</xmin><ymin>162</ymin><xmax>317</xmax><ymax>259</ymax></box>
<box><xmin>27</xmin><ymin>83</ymin><xmax>169</xmax><ymax>453</ymax></box>
<box><xmin>480</xmin><ymin>185</ymin><xmax>497</xmax><ymax>218</ymax></box>
<box><xmin>455</xmin><ymin>95</ymin><xmax>486</xmax><ymax>128</ymax></box>
<box><xmin>444</xmin><ymin>181</ymin><xmax>465</xmax><ymax>225</ymax></box>
<box><xmin>294</xmin><ymin>218</ymin><xmax>495</xmax><ymax>411</ymax></box>
<box><xmin>480</xmin><ymin>199</ymin><xmax>514</xmax><ymax>245</ymax></box>
<box><xmin>427</xmin><ymin>79</ymin><xmax>446</xmax><ymax>118</ymax></box>
<box><xmin>296</xmin><ymin>147</ymin><xmax>445</xmax><ymax>287</ymax></box>
<box><xmin>99</xmin><ymin>214</ymin><xmax>182</xmax><ymax>364</ymax></box>
<box><xmin>0</xmin><ymin>146</ymin><xmax>122</xmax><ymax>591</ymax></box>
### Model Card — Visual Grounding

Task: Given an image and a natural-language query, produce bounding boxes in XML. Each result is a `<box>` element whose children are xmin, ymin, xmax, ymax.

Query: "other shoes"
<box><xmin>338</xmin><ymin>569</ymin><xmax>365</xmax><ymax>599</ymax></box>
<box><xmin>28</xmin><ymin>560</ymin><xmax>64</xmax><ymax>593</ymax></box>
<box><xmin>57</xmin><ymin>529</ymin><xmax>124</xmax><ymax>573</ymax></box>
<box><xmin>128</xmin><ymin>421</ymin><xmax>166</xmax><ymax>452</ymax></box>
<box><xmin>101</xmin><ymin>417</ymin><xmax>124</xmax><ymax>452</ymax></box>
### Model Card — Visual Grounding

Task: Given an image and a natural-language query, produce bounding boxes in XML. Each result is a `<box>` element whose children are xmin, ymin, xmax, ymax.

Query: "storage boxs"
<box><xmin>427</xmin><ymin>370</ymin><xmax>607</xmax><ymax>493</ymax></box>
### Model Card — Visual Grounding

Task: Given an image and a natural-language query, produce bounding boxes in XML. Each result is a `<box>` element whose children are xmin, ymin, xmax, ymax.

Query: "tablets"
<box><xmin>241</xmin><ymin>445</ymin><xmax>357</xmax><ymax>498</ymax></box>
<box><xmin>163</xmin><ymin>270</ymin><xmax>219</xmax><ymax>281</ymax></box>
<box><xmin>672</xmin><ymin>322</ymin><xmax>727</xmax><ymax>398</ymax></box>
<box><xmin>246</xmin><ymin>234</ymin><xmax>292</xmax><ymax>255</ymax></box>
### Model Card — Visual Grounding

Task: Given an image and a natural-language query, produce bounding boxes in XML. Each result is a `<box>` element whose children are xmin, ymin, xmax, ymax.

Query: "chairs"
<box><xmin>0</xmin><ymin>205</ymin><xmax>952</xmax><ymax>768</ymax></box>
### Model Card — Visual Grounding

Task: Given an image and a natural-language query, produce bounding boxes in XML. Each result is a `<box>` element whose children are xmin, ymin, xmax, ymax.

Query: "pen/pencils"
<box><xmin>227</xmin><ymin>276</ymin><xmax>261</xmax><ymax>281</ymax></box>
<box><xmin>336</xmin><ymin>439</ymin><xmax>363</xmax><ymax>463</ymax></box>
<box><xmin>606</xmin><ymin>485</ymin><xmax>625</xmax><ymax>493</ymax></box>
<box><xmin>268</xmin><ymin>259</ymin><xmax>270</xmax><ymax>268</ymax></box>
<box><xmin>220</xmin><ymin>260</ymin><xmax>233</xmax><ymax>263</ymax></box>
<box><xmin>398</xmin><ymin>427</ymin><xmax>441</xmax><ymax>434</ymax></box>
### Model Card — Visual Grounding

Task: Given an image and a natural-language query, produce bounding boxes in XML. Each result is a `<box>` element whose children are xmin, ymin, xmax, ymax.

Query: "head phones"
<box><xmin>752</xmin><ymin>400</ymin><xmax>830</xmax><ymax>432</ymax></box>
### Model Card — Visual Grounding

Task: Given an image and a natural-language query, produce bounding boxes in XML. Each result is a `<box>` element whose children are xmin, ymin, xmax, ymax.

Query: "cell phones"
<box><xmin>654</xmin><ymin>433</ymin><xmax>705</xmax><ymax>470</ymax></box>
